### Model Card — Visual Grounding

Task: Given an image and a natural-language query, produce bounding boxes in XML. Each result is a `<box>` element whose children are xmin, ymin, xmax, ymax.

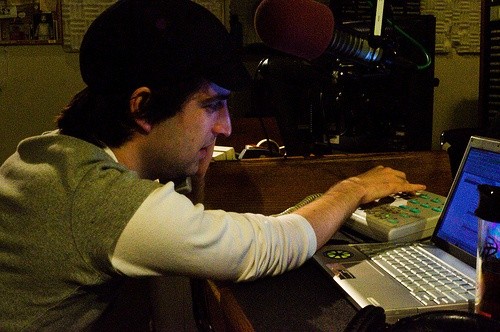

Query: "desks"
<box><xmin>189</xmin><ymin>259</ymin><xmax>359</xmax><ymax>332</ymax></box>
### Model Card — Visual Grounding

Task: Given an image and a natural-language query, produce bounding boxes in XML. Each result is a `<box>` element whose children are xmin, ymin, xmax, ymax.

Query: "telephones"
<box><xmin>158</xmin><ymin>176</ymin><xmax>192</xmax><ymax>194</ymax></box>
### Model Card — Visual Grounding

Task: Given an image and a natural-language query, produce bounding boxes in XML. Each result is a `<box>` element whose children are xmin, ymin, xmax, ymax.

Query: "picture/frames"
<box><xmin>0</xmin><ymin>0</ymin><xmax>65</xmax><ymax>45</ymax></box>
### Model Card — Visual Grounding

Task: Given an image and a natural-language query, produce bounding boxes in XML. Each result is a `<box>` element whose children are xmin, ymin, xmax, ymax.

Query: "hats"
<box><xmin>79</xmin><ymin>0</ymin><xmax>255</xmax><ymax>93</ymax></box>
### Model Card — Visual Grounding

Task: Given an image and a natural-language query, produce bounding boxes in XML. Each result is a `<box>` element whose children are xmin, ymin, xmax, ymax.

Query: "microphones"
<box><xmin>253</xmin><ymin>0</ymin><xmax>417</xmax><ymax>71</ymax></box>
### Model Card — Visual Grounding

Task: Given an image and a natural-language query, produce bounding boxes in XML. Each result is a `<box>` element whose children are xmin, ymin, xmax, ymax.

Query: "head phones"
<box><xmin>344</xmin><ymin>305</ymin><xmax>500</xmax><ymax>332</ymax></box>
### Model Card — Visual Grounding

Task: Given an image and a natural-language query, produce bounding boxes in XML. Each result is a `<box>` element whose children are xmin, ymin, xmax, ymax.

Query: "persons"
<box><xmin>0</xmin><ymin>0</ymin><xmax>426</xmax><ymax>332</ymax></box>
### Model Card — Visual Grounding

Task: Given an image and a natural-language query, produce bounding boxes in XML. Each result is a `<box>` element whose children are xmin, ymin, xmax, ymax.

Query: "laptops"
<box><xmin>311</xmin><ymin>135</ymin><xmax>500</xmax><ymax>324</ymax></box>
